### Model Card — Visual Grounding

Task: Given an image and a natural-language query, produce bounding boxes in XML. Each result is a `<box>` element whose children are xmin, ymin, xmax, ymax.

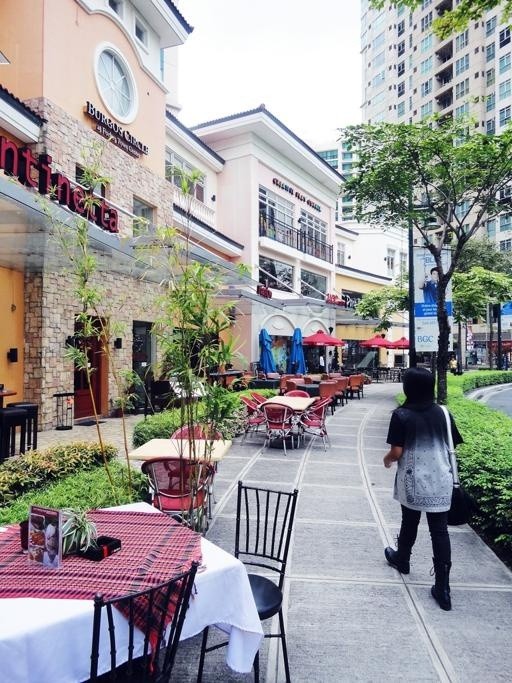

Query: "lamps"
<box><xmin>66</xmin><ymin>336</ymin><xmax>80</xmax><ymax>348</ymax></box>
<box><xmin>114</xmin><ymin>338</ymin><xmax>122</xmax><ymax>348</ymax></box>
<box><xmin>7</xmin><ymin>349</ymin><xmax>17</xmax><ymax>362</ymax></box>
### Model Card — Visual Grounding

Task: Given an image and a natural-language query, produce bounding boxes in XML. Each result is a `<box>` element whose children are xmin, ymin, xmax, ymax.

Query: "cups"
<box><xmin>19</xmin><ymin>520</ymin><xmax>28</xmax><ymax>550</ymax></box>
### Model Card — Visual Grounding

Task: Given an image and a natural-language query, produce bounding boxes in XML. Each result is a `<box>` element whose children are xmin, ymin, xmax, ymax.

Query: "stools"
<box><xmin>1</xmin><ymin>407</ymin><xmax>27</xmax><ymax>465</ymax></box>
<box><xmin>7</xmin><ymin>403</ymin><xmax>38</xmax><ymax>450</ymax></box>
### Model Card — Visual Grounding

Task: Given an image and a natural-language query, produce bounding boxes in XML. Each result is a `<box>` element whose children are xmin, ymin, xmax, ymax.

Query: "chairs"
<box><xmin>90</xmin><ymin>561</ymin><xmax>199</xmax><ymax>683</ymax></box>
<box><xmin>343</xmin><ymin>366</ymin><xmax>405</xmax><ymax>382</ymax></box>
<box><xmin>197</xmin><ymin>482</ymin><xmax>299</xmax><ymax>682</ymax></box>
<box><xmin>240</xmin><ymin>372</ymin><xmax>365</xmax><ymax>455</ymax></box>
<box><xmin>141</xmin><ymin>457</ymin><xmax>215</xmax><ymax>531</ymax></box>
<box><xmin>165</xmin><ymin>424</ymin><xmax>224</xmax><ymax>504</ymax></box>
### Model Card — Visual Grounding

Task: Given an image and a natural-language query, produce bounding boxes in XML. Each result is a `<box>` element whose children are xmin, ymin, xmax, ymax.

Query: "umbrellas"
<box><xmin>358</xmin><ymin>335</ymin><xmax>395</xmax><ymax>367</ymax></box>
<box><xmin>302</xmin><ymin>330</ymin><xmax>345</xmax><ymax>373</ymax></box>
<box><xmin>286</xmin><ymin>328</ymin><xmax>307</xmax><ymax>375</ymax></box>
<box><xmin>387</xmin><ymin>337</ymin><xmax>411</xmax><ymax>369</ymax></box>
<box><xmin>259</xmin><ymin>328</ymin><xmax>277</xmax><ymax>374</ymax></box>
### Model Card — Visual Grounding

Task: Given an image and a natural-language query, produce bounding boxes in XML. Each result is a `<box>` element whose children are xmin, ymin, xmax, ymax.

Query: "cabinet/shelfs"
<box><xmin>55</xmin><ymin>393</ymin><xmax>77</xmax><ymax>428</ymax></box>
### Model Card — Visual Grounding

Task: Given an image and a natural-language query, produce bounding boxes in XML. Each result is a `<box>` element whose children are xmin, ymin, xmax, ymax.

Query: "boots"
<box><xmin>384</xmin><ymin>534</ymin><xmax>416</xmax><ymax>574</ymax></box>
<box><xmin>429</xmin><ymin>557</ymin><xmax>452</xmax><ymax>611</ymax></box>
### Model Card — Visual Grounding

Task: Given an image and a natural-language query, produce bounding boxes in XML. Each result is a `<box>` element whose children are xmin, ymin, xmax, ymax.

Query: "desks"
<box><xmin>209</xmin><ymin>370</ymin><xmax>243</xmax><ymax>388</ymax></box>
<box><xmin>125</xmin><ymin>438</ymin><xmax>233</xmax><ymax>518</ymax></box>
<box><xmin>0</xmin><ymin>389</ymin><xmax>16</xmax><ymax>408</ymax></box>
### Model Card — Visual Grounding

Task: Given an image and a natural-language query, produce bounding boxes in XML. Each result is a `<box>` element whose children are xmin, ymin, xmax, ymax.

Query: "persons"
<box><xmin>384</xmin><ymin>368</ymin><xmax>464</xmax><ymax>611</ymax></box>
<box><xmin>419</xmin><ymin>267</ymin><xmax>441</xmax><ymax>303</ymax></box>
<box><xmin>320</xmin><ymin>353</ymin><xmax>325</xmax><ymax>373</ymax></box>
<box><xmin>449</xmin><ymin>355</ymin><xmax>458</xmax><ymax>376</ymax></box>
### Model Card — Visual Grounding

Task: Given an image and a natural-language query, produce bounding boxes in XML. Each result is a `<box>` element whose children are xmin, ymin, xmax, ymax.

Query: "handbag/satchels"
<box><xmin>446</xmin><ymin>483</ymin><xmax>480</xmax><ymax>526</ymax></box>
